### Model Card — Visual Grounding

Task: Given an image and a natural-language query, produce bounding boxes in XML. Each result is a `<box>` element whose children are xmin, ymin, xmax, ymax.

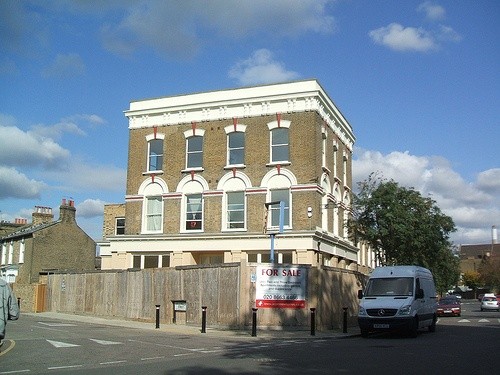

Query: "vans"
<box><xmin>356</xmin><ymin>265</ymin><xmax>438</xmax><ymax>339</ymax></box>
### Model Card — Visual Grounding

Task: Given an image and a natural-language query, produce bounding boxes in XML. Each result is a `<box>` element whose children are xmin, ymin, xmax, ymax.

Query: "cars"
<box><xmin>480</xmin><ymin>293</ymin><xmax>499</xmax><ymax>312</ymax></box>
<box><xmin>434</xmin><ymin>296</ymin><xmax>461</xmax><ymax>317</ymax></box>
